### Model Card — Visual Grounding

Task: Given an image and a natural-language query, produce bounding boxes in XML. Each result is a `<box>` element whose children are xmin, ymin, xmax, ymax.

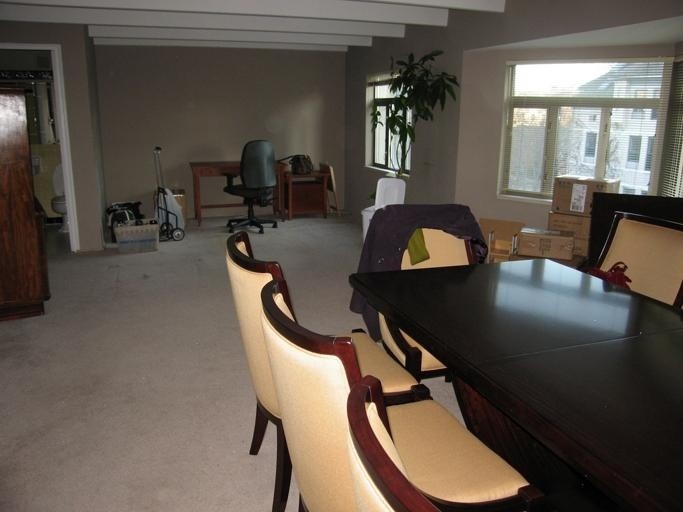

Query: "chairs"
<box><xmin>347</xmin><ymin>375</ymin><xmax>542</xmax><ymax>512</ymax></box>
<box><xmin>259</xmin><ymin>279</ymin><xmax>543</xmax><ymax>512</ymax></box>
<box><xmin>225</xmin><ymin>230</ymin><xmax>420</xmax><ymax>512</ymax></box>
<box><xmin>223</xmin><ymin>139</ymin><xmax>277</xmax><ymax>234</ymax></box>
<box><xmin>595</xmin><ymin>211</ymin><xmax>683</xmax><ymax>309</ymax></box>
<box><xmin>362</xmin><ymin>204</ymin><xmax>479</xmax><ymax>383</ymax></box>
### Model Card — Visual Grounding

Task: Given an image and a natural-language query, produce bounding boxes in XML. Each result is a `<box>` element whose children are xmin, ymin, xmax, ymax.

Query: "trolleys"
<box><xmin>152</xmin><ymin>146</ymin><xmax>186</xmax><ymax>243</ymax></box>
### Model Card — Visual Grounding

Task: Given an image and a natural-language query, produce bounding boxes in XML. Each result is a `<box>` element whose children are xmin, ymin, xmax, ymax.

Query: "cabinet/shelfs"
<box><xmin>0</xmin><ymin>94</ymin><xmax>50</xmax><ymax>320</ymax></box>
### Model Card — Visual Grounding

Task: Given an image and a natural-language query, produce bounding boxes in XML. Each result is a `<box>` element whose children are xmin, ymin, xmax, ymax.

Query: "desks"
<box><xmin>188</xmin><ymin>161</ymin><xmax>289</xmax><ymax>226</ymax></box>
<box><xmin>349</xmin><ymin>258</ymin><xmax>682</xmax><ymax>512</ymax></box>
<box><xmin>273</xmin><ymin>170</ymin><xmax>330</xmax><ymax>220</ymax></box>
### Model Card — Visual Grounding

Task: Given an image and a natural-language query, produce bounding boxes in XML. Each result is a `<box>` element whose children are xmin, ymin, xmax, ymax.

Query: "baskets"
<box><xmin>113</xmin><ymin>219</ymin><xmax>159</xmax><ymax>241</ymax></box>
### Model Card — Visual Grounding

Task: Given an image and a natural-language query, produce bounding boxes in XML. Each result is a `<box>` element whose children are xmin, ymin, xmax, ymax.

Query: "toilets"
<box><xmin>51</xmin><ymin>163</ymin><xmax>69</xmax><ymax>233</ymax></box>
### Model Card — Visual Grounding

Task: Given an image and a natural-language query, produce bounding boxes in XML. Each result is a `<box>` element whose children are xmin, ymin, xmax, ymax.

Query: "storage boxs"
<box><xmin>518</xmin><ymin>226</ymin><xmax>576</xmax><ymax>260</ymax></box>
<box><xmin>548</xmin><ymin>210</ymin><xmax>591</xmax><ymax>257</ymax></box>
<box><xmin>551</xmin><ymin>178</ymin><xmax>620</xmax><ymax>219</ymax></box>
<box><xmin>479</xmin><ymin>218</ymin><xmax>525</xmax><ymax>252</ymax></box>
<box><xmin>113</xmin><ymin>218</ymin><xmax>160</xmax><ymax>254</ymax></box>
